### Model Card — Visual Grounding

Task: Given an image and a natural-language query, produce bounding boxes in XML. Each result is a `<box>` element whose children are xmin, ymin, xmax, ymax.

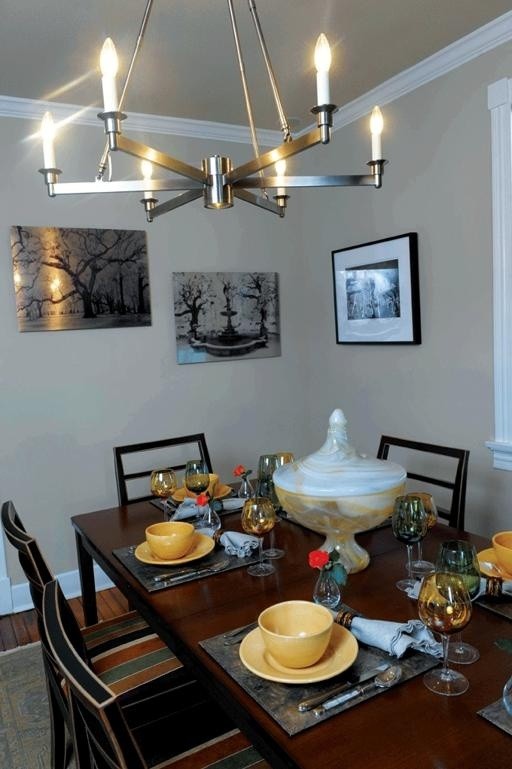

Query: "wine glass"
<box><xmin>388</xmin><ymin>492</ymin><xmax>428</xmax><ymax>598</ymax></box>
<box><xmin>184</xmin><ymin>456</ymin><xmax>211</xmax><ymax>532</ymax></box>
<box><xmin>433</xmin><ymin>538</ymin><xmax>485</xmax><ymax>669</ymax></box>
<box><xmin>240</xmin><ymin>492</ymin><xmax>278</xmax><ymax>583</ymax></box>
<box><xmin>254</xmin><ymin>452</ymin><xmax>277</xmax><ymax>514</ymax></box>
<box><xmin>413</xmin><ymin>569</ymin><xmax>477</xmax><ymax>700</ymax></box>
<box><xmin>256</xmin><ymin>475</ymin><xmax>291</xmax><ymax>564</ymax></box>
<box><xmin>147</xmin><ymin>466</ymin><xmax>177</xmax><ymax>525</ymax></box>
<box><xmin>401</xmin><ymin>488</ymin><xmax>438</xmax><ymax>577</ymax></box>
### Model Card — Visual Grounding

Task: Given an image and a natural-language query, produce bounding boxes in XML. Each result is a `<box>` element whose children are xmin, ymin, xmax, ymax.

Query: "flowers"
<box><xmin>233</xmin><ymin>461</ymin><xmax>254</xmax><ymax>492</ymax></box>
<box><xmin>307</xmin><ymin>550</ymin><xmax>347</xmax><ymax>587</ymax></box>
<box><xmin>196</xmin><ymin>493</ymin><xmax>224</xmax><ymax>520</ymax></box>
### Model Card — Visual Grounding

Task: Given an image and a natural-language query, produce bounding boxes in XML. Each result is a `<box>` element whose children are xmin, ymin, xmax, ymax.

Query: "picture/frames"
<box><xmin>332</xmin><ymin>231</ymin><xmax>422</xmax><ymax>345</ymax></box>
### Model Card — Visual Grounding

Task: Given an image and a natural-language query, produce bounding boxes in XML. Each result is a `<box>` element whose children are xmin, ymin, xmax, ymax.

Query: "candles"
<box><xmin>271</xmin><ymin>149</ymin><xmax>289</xmax><ymax>197</ymax></box>
<box><xmin>36</xmin><ymin>108</ymin><xmax>62</xmax><ymax>168</ymax></box>
<box><xmin>94</xmin><ymin>33</ymin><xmax>122</xmax><ymax>110</ymax></box>
<box><xmin>137</xmin><ymin>153</ymin><xmax>158</xmax><ymax>197</ymax></box>
<box><xmin>367</xmin><ymin>103</ymin><xmax>388</xmax><ymax>164</ymax></box>
<box><xmin>310</xmin><ymin>28</ymin><xmax>336</xmax><ymax>105</ymax></box>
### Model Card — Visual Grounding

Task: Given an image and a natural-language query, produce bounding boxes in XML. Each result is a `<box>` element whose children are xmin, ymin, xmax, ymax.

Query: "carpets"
<box><xmin>3</xmin><ymin>624</ymin><xmax>118</xmax><ymax>767</ymax></box>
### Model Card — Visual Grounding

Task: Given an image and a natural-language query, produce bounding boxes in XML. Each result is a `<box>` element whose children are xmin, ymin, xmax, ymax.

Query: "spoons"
<box><xmin>164</xmin><ymin>558</ymin><xmax>235</xmax><ymax>586</ymax></box>
<box><xmin>311</xmin><ymin>661</ymin><xmax>412</xmax><ymax>720</ymax></box>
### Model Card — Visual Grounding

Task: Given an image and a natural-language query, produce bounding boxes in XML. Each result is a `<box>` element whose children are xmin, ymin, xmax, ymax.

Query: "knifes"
<box><xmin>296</xmin><ymin>659</ymin><xmax>391</xmax><ymax>714</ymax></box>
<box><xmin>150</xmin><ymin>553</ymin><xmax>221</xmax><ymax>586</ymax></box>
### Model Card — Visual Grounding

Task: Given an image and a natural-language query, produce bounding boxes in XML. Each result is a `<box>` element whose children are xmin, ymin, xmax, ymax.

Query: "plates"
<box><xmin>238</xmin><ymin>621</ymin><xmax>363</xmax><ymax>690</ymax></box>
<box><xmin>166</xmin><ymin>482</ymin><xmax>235</xmax><ymax>504</ymax></box>
<box><xmin>130</xmin><ymin>533</ymin><xmax>220</xmax><ymax>568</ymax></box>
<box><xmin>469</xmin><ymin>543</ymin><xmax>512</xmax><ymax>582</ymax></box>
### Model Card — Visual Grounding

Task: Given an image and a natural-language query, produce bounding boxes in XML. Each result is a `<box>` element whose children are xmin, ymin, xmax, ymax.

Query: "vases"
<box><xmin>312</xmin><ymin>570</ymin><xmax>341</xmax><ymax>607</ymax></box>
<box><xmin>239</xmin><ymin>476</ymin><xmax>254</xmax><ymax>502</ymax></box>
<box><xmin>200</xmin><ymin>505</ymin><xmax>222</xmax><ymax>531</ymax></box>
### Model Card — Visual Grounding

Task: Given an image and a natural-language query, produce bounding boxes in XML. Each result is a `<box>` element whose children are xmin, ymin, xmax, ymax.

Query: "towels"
<box><xmin>329</xmin><ymin>612</ymin><xmax>445</xmax><ymax>659</ymax></box>
<box><xmin>169</xmin><ymin>496</ymin><xmax>248</xmax><ymax>523</ymax></box>
<box><xmin>194</xmin><ymin>527</ymin><xmax>261</xmax><ymax>558</ymax></box>
<box><xmin>409</xmin><ymin>569</ymin><xmax>512</xmax><ymax>604</ymax></box>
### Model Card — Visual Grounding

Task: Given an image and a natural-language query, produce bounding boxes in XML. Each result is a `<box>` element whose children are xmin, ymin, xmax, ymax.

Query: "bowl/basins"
<box><xmin>255</xmin><ymin>598</ymin><xmax>334</xmax><ymax>670</ymax></box>
<box><xmin>491</xmin><ymin>523</ymin><xmax>512</xmax><ymax>579</ymax></box>
<box><xmin>180</xmin><ymin>470</ymin><xmax>222</xmax><ymax>496</ymax></box>
<box><xmin>142</xmin><ymin>518</ymin><xmax>197</xmax><ymax>565</ymax></box>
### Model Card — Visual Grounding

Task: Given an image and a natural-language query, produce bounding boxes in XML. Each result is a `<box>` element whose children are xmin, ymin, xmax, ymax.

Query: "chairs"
<box><xmin>39</xmin><ymin>578</ymin><xmax>272</xmax><ymax>767</ymax></box>
<box><xmin>1</xmin><ymin>502</ymin><xmax>215</xmax><ymax>769</ymax></box>
<box><xmin>113</xmin><ymin>433</ymin><xmax>213</xmax><ymax>614</ymax></box>
<box><xmin>376</xmin><ymin>435</ymin><xmax>471</xmax><ymax>532</ymax></box>
